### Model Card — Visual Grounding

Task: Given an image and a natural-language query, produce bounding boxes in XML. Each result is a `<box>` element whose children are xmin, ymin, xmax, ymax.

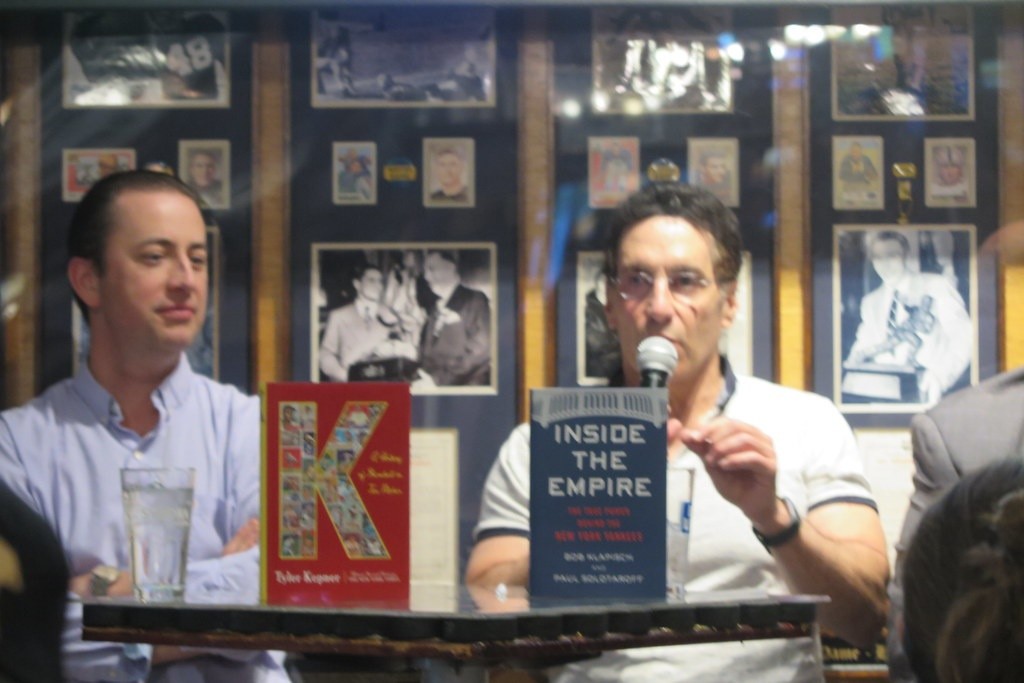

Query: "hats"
<box><xmin>337</xmin><ymin>148</ymin><xmax>371</xmax><ymax>166</ymax></box>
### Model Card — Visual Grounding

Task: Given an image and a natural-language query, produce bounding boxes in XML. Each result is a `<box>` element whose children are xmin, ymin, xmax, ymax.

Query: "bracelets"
<box><xmin>752</xmin><ymin>498</ymin><xmax>801</xmax><ymax>554</ymax></box>
<box><xmin>89</xmin><ymin>564</ymin><xmax>118</xmax><ymax>599</ymax></box>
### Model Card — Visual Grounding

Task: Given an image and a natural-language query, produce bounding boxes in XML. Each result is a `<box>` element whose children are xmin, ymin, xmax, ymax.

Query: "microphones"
<box><xmin>636</xmin><ymin>335</ymin><xmax>678</xmax><ymax>387</ymax></box>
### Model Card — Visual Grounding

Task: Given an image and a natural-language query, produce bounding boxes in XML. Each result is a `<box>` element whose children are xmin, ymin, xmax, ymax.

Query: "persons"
<box><xmin>187</xmin><ymin>150</ymin><xmax>222</xmax><ymax>204</ymax></box>
<box><xmin>429</xmin><ymin>146</ymin><xmax>467</xmax><ymax>204</ymax></box>
<box><xmin>0</xmin><ymin>170</ymin><xmax>290</xmax><ymax>683</ymax></box>
<box><xmin>318</xmin><ymin>249</ymin><xmax>491</xmax><ymax>386</ymax></box>
<box><xmin>464</xmin><ymin>181</ymin><xmax>890</xmax><ymax>683</ymax></box>
<box><xmin>585</xmin><ymin>269</ymin><xmax>623</xmax><ymax>378</ymax></box>
<box><xmin>838</xmin><ymin>228</ymin><xmax>972</xmax><ymax>402</ymax></box>
<box><xmin>890</xmin><ymin>368</ymin><xmax>1024</xmax><ymax>683</ymax></box>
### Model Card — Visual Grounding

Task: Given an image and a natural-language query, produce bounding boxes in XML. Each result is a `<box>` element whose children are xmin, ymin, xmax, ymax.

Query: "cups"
<box><xmin>120</xmin><ymin>465</ymin><xmax>195</xmax><ymax>596</ymax></box>
<box><xmin>666</xmin><ymin>464</ymin><xmax>697</xmax><ymax>599</ymax></box>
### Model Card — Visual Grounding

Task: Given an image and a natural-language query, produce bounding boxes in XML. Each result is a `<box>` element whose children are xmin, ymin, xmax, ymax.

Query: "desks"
<box><xmin>79</xmin><ymin>588</ymin><xmax>831</xmax><ymax>683</ymax></box>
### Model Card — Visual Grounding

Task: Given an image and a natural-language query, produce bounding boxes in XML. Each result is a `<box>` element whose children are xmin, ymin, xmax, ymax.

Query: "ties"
<box><xmin>887</xmin><ymin>291</ymin><xmax>899</xmax><ymax>356</ymax></box>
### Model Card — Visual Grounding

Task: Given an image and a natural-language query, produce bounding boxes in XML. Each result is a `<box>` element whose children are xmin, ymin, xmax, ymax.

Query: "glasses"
<box><xmin>609</xmin><ymin>268</ymin><xmax>718</xmax><ymax>302</ymax></box>
